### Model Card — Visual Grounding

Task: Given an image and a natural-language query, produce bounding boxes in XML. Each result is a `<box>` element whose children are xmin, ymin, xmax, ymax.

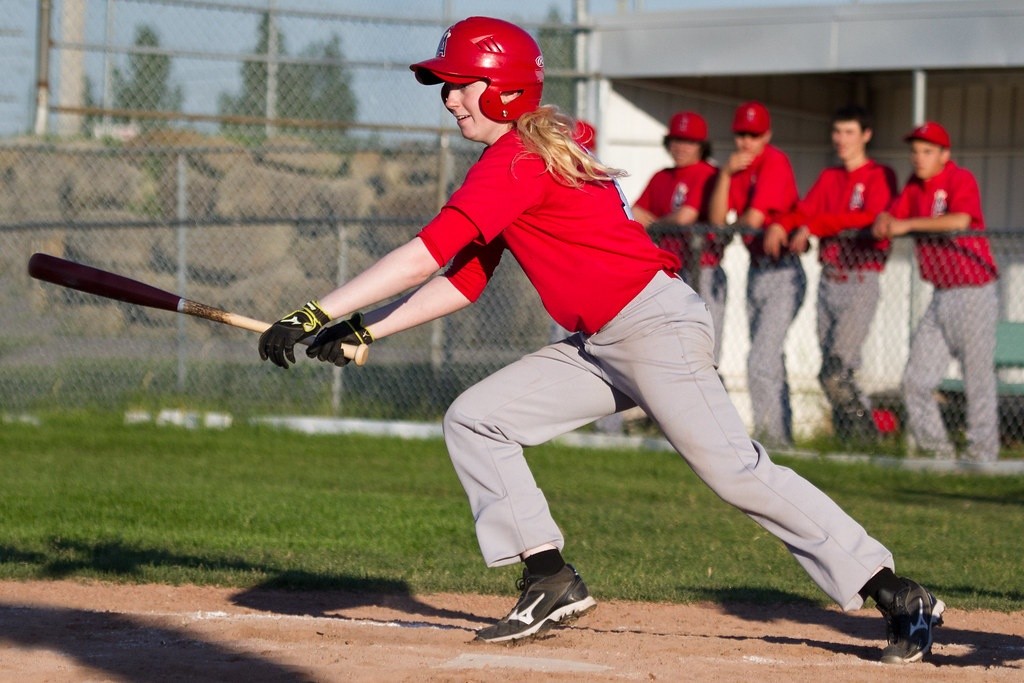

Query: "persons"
<box><xmin>631</xmin><ymin>112</ymin><xmax>726</xmax><ymax>371</ymax></box>
<box><xmin>709</xmin><ymin>101</ymin><xmax>807</xmax><ymax>448</ymax></box>
<box><xmin>259</xmin><ymin>17</ymin><xmax>946</xmax><ymax>664</ymax></box>
<box><xmin>763</xmin><ymin>106</ymin><xmax>898</xmax><ymax>439</ymax></box>
<box><xmin>871</xmin><ymin>121</ymin><xmax>1001</xmax><ymax>462</ymax></box>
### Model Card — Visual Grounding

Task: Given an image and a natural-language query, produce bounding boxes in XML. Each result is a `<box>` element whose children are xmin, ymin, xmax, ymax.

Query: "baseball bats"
<box><xmin>25</xmin><ymin>250</ymin><xmax>370</xmax><ymax>365</ymax></box>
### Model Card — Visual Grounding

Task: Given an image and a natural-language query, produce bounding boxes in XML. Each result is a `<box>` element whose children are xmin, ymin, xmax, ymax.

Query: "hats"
<box><xmin>904</xmin><ymin>122</ymin><xmax>951</xmax><ymax>150</ymax></box>
<box><xmin>669</xmin><ymin>112</ymin><xmax>708</xmax><ymax>141</ymax></box>
<box><xmin>732</xmin><ymin>102</ymin><xmax>771</xmax><ymax>135</ymax></box>
<box><xmin>562</xmin><ymin>120</ymin><xmax>599</xmax><ymax>152</ymax></box>
<box><xmin>415</xmin><ymin>67</ymin><xmax>482</xmax><ymax>85</ymax></box>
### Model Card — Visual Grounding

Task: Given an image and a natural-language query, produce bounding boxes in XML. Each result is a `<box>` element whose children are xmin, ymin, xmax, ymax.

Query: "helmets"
<box><xmin>410</xmin><ymin>16</ymin><xmax>544</xmax><ymax>122</ymax></box>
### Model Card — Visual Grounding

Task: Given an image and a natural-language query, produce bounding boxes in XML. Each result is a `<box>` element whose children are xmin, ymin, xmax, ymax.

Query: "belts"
<box><xmin>752</xmin><ymin>256</ymin><xmax>798</xmax><ymax>269</ymax></box>
<box><xmin>581</xmin><ymin>270</ymin><xmax>682</xmax><ymax>340</ymax></box>
<box><xmin>823</xmin><ymin>268</ymin><xmax>865</xmax><ymax>286</ymax></box>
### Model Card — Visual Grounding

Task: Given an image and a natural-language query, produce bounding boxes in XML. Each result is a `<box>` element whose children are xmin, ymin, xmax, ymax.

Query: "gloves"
<box><xmin>306</xmin><ymin>312</ymin><xmax>376</xmax><ymax>367</ymax></box>
<box><xmin>258</xmin><ymin>299</ymin><xmax>331</xmax><ymax>370</ymax></box>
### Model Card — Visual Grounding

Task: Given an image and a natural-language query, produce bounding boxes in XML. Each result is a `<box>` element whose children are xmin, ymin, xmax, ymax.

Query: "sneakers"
<box><xmin>875</xmin><ymin>576</ymin><xmax>947</xmax><ymax>664</ymax></box>
<box><xmin>475</xmin><ymin>564</ymin><xmax>597</xmax><ymax>642</ymax></box>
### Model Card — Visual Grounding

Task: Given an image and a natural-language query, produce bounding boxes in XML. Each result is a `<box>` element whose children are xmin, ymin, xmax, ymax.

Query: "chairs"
<box><xmin>943</xmin><ymin>321</ymin><xmax>1024</xmax><ymax>448</ymax></box>
